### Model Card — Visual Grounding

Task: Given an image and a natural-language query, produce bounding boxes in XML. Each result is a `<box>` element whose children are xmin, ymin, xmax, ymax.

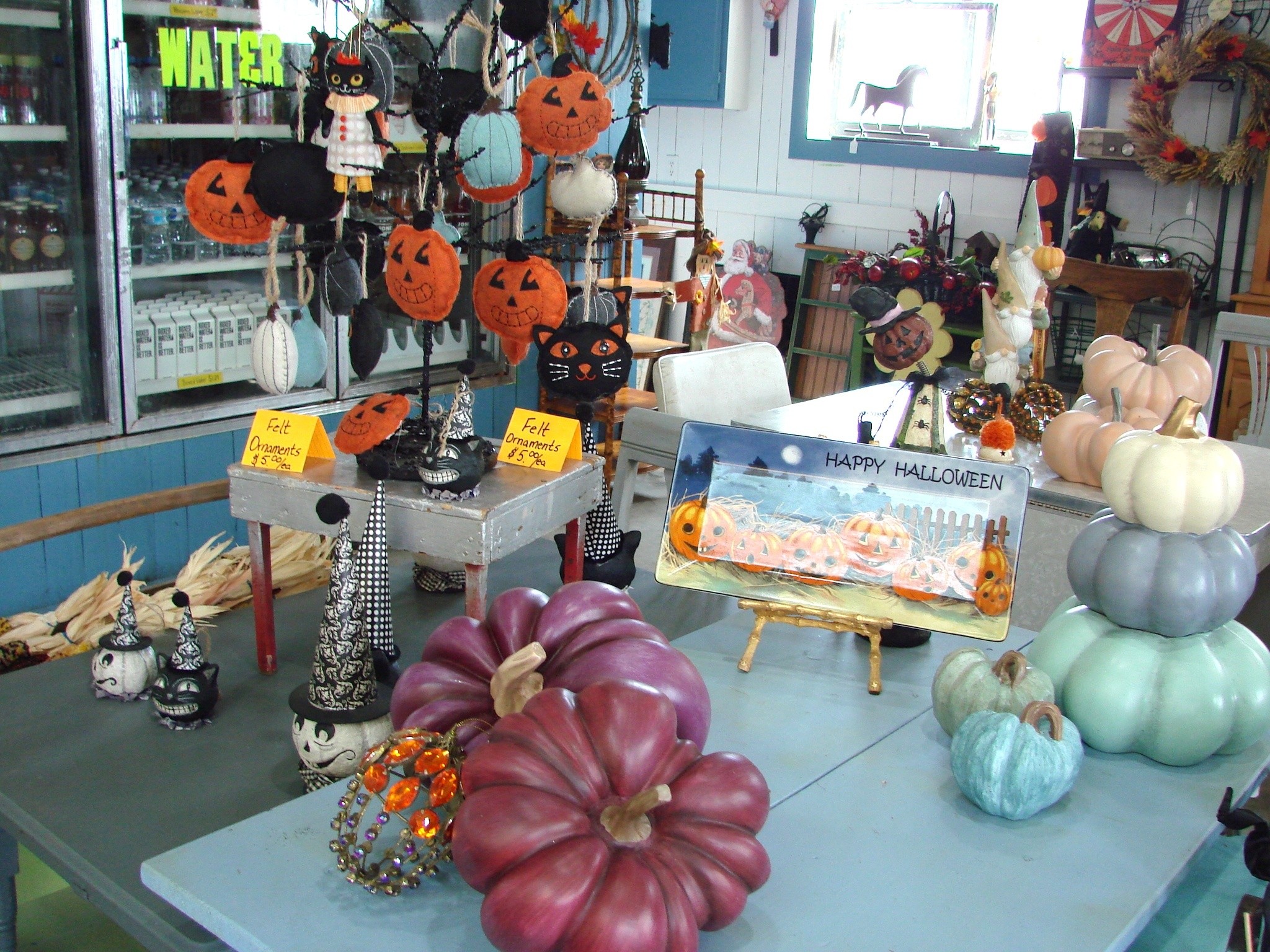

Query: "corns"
<box><xmin>0</xmin><ymin>616</ymin><xmax>85</xmax><ymax>675</ymax></box>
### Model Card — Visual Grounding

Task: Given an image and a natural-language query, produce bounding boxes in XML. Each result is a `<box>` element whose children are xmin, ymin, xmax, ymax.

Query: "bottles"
<box><xmin>0</xmin><ymin>55</ymin><xmax>67</xmax><ymax>126</ymax></box>
<box><xmin>127</xmin><ymin>55</ymin><xmax>273</xmax><ymax>126</ymax></box>
<box><xmin>0</xmin><ymin>160</ymin><xmax>70</xmax><ymax>275</ymax></box>
<box><xmin>128</xmin><ymin>156</ymin><xmax>268</xmax><ymax>266</ymax></box>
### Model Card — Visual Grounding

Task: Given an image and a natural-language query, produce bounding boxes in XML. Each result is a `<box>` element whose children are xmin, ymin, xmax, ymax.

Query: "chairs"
<box><xmin>653</xmin><ymin>342</ymin><xmax>791</xmax><ymax>425</ymax></box>
<box><xmin>1200</xmin><ymin>311</ymin><xmax>1270</xmax><ymax>450</ymax></box>
<box><xmin>1031</xmin><ymin>254</ymin><xmax>1195</xmax><ymax>385</ymax></box>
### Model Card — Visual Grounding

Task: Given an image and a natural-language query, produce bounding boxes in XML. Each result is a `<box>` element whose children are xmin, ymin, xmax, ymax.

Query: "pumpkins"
<box><xmin>932</xmin><ymin>324</ymin><xmax>1269</xmax><ymax>821</ymax></box>
<box><xmin>389</xmin><ymin>580</ymin><xmax>770</xmax><ymax>952</ymax></box>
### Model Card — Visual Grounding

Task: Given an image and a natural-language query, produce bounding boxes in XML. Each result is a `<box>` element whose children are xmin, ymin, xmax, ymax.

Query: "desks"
<box><xmin>1</xmin><ymin>381</ymin><xmax>1270</xmax><ymax>952</ymax></box>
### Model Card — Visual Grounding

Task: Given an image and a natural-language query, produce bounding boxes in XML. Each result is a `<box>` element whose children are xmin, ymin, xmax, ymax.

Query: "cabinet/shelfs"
<box><xmin>538</xmin><ymin>162</ymin><xmax>704</xmax><ymax>491</ymax></box>
<box><xmin>0</xmin><ymin>0</ymin><xmax>522</xmax><ymax>457</ymax></box>
<box><xmin>1054</xmin><ymin>58</ymin><xmax>1270</xmax><ymax>441</ymax></box>
